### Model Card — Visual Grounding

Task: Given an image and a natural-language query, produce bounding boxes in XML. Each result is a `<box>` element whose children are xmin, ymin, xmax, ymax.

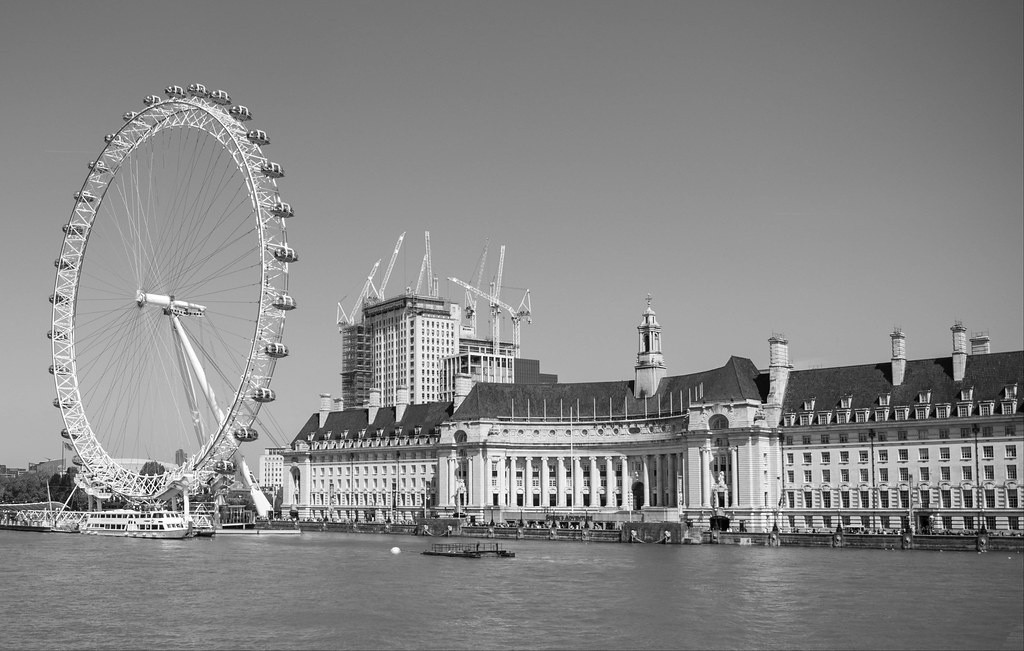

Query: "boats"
<box><xmin>78</xmin><ymin>506</ymin><xmax>190</xmax><ymax>541</ymax></box>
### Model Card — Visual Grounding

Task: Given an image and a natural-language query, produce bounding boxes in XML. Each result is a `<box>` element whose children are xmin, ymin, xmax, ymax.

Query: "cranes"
<box><xmin>334</xmin><ymin>230</ymin><xmax>535</xmax><ymax>357</ymax></box>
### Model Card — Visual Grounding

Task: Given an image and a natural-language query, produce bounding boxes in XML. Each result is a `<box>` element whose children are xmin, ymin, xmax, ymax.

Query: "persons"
<box><xmin>494</xmin><ymin>522</ymin><xmax>519</xmax><ymax>527</ymax></box>
<box><xmin>779</xmin><ymin>528</ymin><xmax>782</xmax><ymax>533</ymax></box>
<box><xmin>256</xmin><ymin>516</ymin><xmax>369</xmax><ymax>523</ymax></box>
<box><xmin>988</xmin><ymin>531</ymin><xmax>1021</xmax><ymax>536</ymax></box>
<box><xmin>792</xmin><ymin>527</ymin><xmax>833</xmax><ymax>533</ymax></box>
<box><xmin>595</xmin><ymin>524</ymin><xmax>602</xmax><ymax>529</ymax></box>
<box><xmin>526</xmin><ymin>522</ymin><xmax>551</xmax><ymax>528</ymax></box>
<box><xmin>916</xmin><ymin>527</ymin><xmax>978</xmax><ymax>535</ymax></box>
<box><xmin>467</xmin><ymin>521</ymin><xmax>489</xmax><ymax>527</ymax></box>
<box><xmin>393</xmin><ymin>519</ymin><xmax>415</xmax><ymax>524</ymax></box>
<box><xmin>719</xmin><ymin>527</ymin><xmax>747</xmax><ymax>533</ymax></box>
<box><xmin>558</xmin><ymin>524</ymin><xmax>584</xmax><ymax>529</ymax></box>
<box><xmin>850</xmin><ymin>528</ymin><xmax>906</xmax><ymax>535</ymax></box>
<box><xmin>763</xmin><ymin>527</ymin><xmax>768</xmax><ymax>533</ymax></box>
